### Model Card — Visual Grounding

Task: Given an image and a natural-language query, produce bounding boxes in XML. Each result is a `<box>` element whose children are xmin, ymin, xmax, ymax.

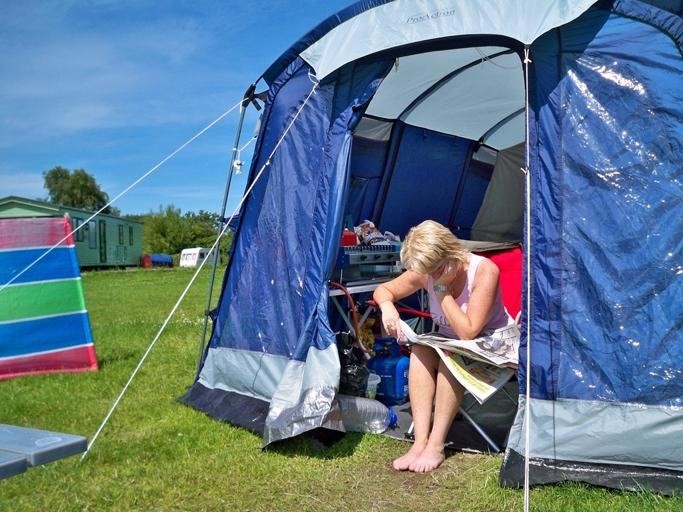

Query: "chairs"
<box><xmin>400</xmin><ymin>247</ymin><xmax>523</xmax><ymax>454</ymax></box>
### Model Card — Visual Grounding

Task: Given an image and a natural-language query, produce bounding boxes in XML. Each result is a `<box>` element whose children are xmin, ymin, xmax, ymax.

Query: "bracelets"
<box><xmin>432</xmin><ymin>283</ymin><xmax>451</xmax><ymax>293</ymax></box>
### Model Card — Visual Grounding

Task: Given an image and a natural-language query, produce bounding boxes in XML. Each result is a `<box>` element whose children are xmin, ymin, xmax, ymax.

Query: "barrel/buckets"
<box><xmin>321</xmin><ymin>395</ymin><xmax>396</xmax><ymax>435</ymax></box>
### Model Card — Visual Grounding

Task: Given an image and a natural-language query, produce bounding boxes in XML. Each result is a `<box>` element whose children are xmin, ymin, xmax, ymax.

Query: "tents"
<box><xmin>173</xmin><ymin>1</ymin><xmax>682</xmax><ymax>500</ymax></box>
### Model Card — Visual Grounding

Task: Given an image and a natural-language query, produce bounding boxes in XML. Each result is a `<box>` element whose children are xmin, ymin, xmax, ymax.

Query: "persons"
<box><xmin>372</xmin><ymin>219</ymin><xmax>514</xmax><ymax>473</ymax></box>
